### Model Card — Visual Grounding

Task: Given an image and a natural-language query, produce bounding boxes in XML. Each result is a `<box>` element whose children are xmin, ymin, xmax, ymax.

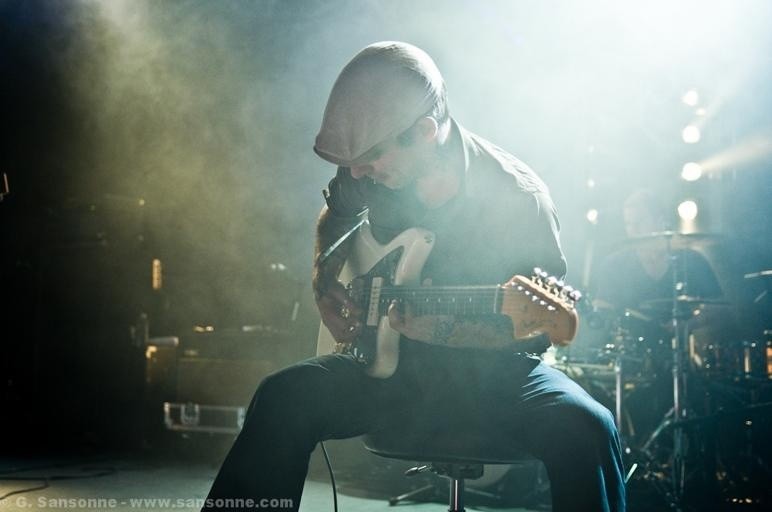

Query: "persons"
<box><xmin>587</xmin><ymin>186</ymin><xmax>744</xmax><ymax>512</ymax></box>
<box><xmin>197</xmin><ymin>39</ymin><xmax>626</xmax><ymax>512</ymax></box>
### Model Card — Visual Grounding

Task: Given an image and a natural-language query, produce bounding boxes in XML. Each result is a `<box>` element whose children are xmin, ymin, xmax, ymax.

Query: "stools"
<box><xmin>365</xmin><ymin>425</ymin><xmax>540</xmax><ymax>512</ymax></box>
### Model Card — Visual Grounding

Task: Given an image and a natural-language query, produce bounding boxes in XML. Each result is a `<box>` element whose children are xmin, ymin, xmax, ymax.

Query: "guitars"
<box><xmin>316</xmin><ymin>221</ymin><xmax>582</xmax><ymax>378</ymax></box>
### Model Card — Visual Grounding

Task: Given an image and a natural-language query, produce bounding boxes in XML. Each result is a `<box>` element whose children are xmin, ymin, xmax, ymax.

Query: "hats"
<box><xmin>312</xmin><ymin>38</ymin><xmax>444</xmax><ymax>168</ymax></box>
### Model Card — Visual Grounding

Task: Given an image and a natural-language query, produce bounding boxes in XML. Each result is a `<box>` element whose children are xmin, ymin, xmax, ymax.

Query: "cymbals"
<box><xmin>637</xmin><ymin>298</ymin><xmax>730</xmax><ymax>306</ymax></box>
<box><xmin>624</xmin><ymin>233</ymin><xmax>724</xmax><ymax>248</ymax></box>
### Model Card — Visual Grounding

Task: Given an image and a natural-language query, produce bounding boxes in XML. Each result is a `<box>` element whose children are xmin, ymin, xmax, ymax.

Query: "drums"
<box><xmin>700</xmin><ymin>342</ymin><xmax>772</xmax><ymax>383</ymax></box>
<box><xmin>453</xmin><ymin>461</ymin><xmax>546</xmax><ymax>495</ymax></box>
